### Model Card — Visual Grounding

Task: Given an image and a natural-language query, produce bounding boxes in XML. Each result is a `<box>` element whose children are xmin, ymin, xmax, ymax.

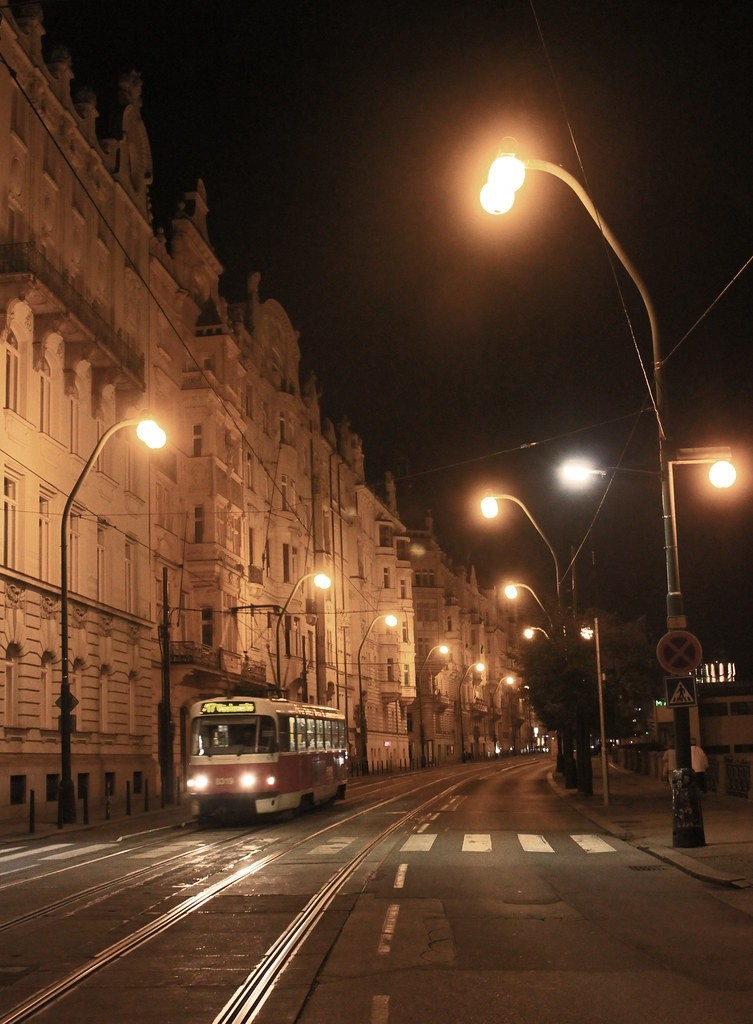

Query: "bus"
<box><xmin>185</xmin><ymin>696</ymin><xmax>350</xmax><ymax>827</ymax></box>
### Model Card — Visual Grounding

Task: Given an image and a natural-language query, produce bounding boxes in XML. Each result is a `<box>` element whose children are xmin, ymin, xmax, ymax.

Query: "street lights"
<box><xmin>523</xmin><ymin>626</ymin><xmax>550</xmax><ymax>643</ymax></box>
<box><xmin>482</xmin><ymin>494</ymin><xmax>561</xmax><ymax>600</ymax></box>
<box><xmin>418</xmin><ymin>645</ymin><xmax>449</xmax><ymax>769</ymax></box>
<box><xmin>478</xmin><ymin>150</ymin><xmax>736</xmax><ymax>850</ymax></box>
<box><xmin>357</xmin><ymin>614</ymin><xmax>398</xmax><ymax>775</ymax></box>
<box><xmin>492</xmin><ymin>676</ymin><xmax>514</xmax><ymax>760</ymax></box>
<box><xmin>275</xmin><ymin>571</ymin><xmax>332</xmax><ymax>696</ymax></box>
<box><xmin>459</xmin><ymin>663</ymin><xmax>484</xmax><ymax>763</ymax></box>
<box><xmin>503</xmin><ymin>584</ymin><xmax>553</xmax><ymax>629</ymax></box>
<box><xmin>56</xmin><ymin>416</ymin><xmax>168</xmax><ymax>823</ymax></box>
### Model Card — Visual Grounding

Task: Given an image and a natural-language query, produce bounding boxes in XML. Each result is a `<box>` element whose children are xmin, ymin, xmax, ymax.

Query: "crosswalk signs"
<box><xmin>665</xmin><ymin>677</ymin><xmax>698</xmax><ymax>708</ymax></box>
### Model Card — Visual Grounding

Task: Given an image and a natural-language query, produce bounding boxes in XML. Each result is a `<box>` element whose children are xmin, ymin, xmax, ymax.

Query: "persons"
<box><xmin>662</xmin><ymin>740</ymin><xmax>677</xmax><ymax>811</ymax></box>
<box><xmin>689</xmin><ymin>737</ymin><xmax>710</xmax><ymax>816</ymax></box>
